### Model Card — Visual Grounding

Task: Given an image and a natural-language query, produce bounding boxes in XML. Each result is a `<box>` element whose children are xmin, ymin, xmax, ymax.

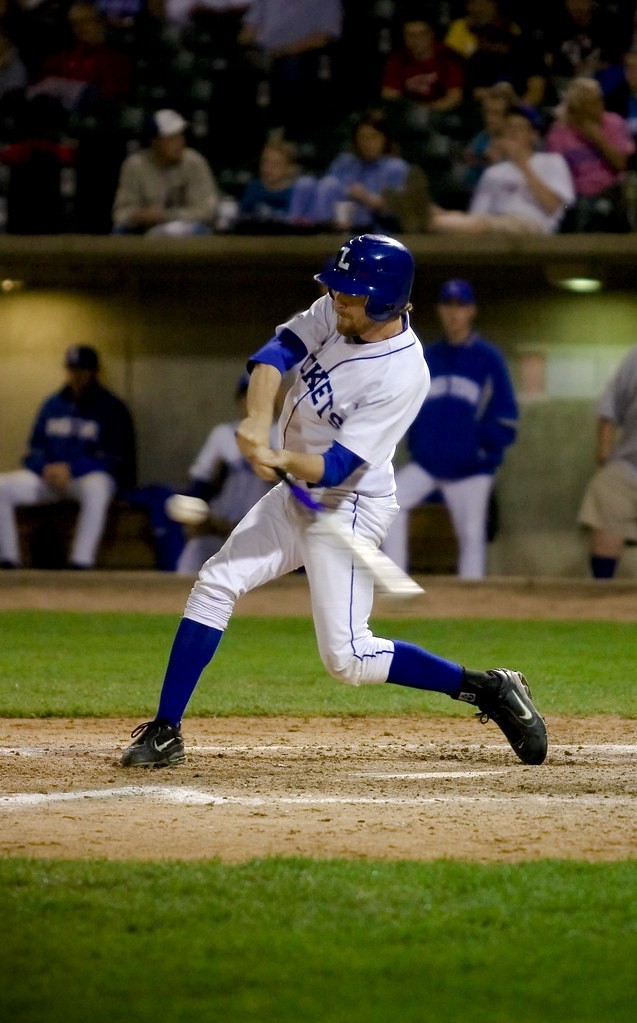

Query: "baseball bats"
<box><xmin>273</xmin><ymin>467</ymin><xmax>427</xmax><ymax>597</ymax></box>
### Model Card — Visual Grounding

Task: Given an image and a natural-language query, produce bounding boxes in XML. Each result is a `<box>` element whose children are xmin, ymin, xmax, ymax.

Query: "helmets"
<box><xmin>64</xmin><ymin>345</ymin><xmax>101</xmax><ymax>374</ymax></box>
<box><xmin>313</xmin><ymin>234</ymin><xmax>413</xmax><ymax>322</ymax></box>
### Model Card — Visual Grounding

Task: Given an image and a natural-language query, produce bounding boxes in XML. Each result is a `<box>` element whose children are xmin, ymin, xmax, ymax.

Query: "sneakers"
<box><xmin>474</xmin><ymin>667</ymin><xmax>547</xmax><ymax>765</ymax></box>
<box><xmin>122</xmin><ymin>721</ymin><xmax>186</xmax><ymax>768</ymax></box>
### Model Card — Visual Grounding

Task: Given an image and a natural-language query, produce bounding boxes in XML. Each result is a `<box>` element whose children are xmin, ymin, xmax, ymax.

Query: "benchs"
<box><xmin>14</xmin><ymin>502</ymin><xmax>456</xmax><ymax>571</ymax></box>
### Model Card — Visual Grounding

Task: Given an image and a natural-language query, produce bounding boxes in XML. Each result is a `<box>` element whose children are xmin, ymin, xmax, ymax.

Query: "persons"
<box><xmin>229</xmin><ymin>138</ymin><xmax>309</xmax><ymax>234</ymax></box>
<box><xmin>1</xmin><ymin>345</ymin><xmax>140</xmax><ymax>572</ymax></box>
<box><xmin>119</xmin><ymin>236</ymin><xmax>547</xmax><ymax>771</ymax></box>
<box><xmin>284</xmin><ymin>124</ymin><xmax>412</xmax><ymax>241</ymax></box>
<box><xmin>114</xmin><ymin>106</ymin><xmax>222</xmax><ymax>239</ymax></box>
<box><xmin>430</xmin><ymin>103</ymin><xmax>576</xmax><ymax>238</ymax></box>
<box><xmin>394</xmin><ymin>278</ymin><xmax>521</xmax><ymax>581</ymax></box>
<box><xmin>0</xmin><ymin>0</ymin><xmax>637</xmax><ymax>237</ymax></box>
<box><xmin>575</xmin><ymin>348</ymin><xmax>637</xmax><ymax>579</ymax></box>
<box><xmin>545</xmin><ymin>76</ymin><xmax>635</xmax><ymax>233</ymax></box>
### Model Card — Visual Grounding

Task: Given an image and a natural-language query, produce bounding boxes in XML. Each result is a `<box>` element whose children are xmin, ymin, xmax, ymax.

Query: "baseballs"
<box><xmin>164</xmin><ymin>494</ymin><xmax>211</xmax><ymax>526</ymax></box>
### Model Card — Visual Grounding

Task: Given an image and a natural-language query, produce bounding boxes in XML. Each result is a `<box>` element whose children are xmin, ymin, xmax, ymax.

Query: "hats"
<box><xmin>150</xmin><ymin>109</ymin><xmax>186</xmax><ymax>139</ymax></box>
<box><xmin>439</xmin><ymin>279</ymin><xmax>475</xmax><ymax>305</ymax></box>
<box><xmin>235</xmin><ymin>373</ymin><xmax>249</xmax><ymax>397</ymax></box>
